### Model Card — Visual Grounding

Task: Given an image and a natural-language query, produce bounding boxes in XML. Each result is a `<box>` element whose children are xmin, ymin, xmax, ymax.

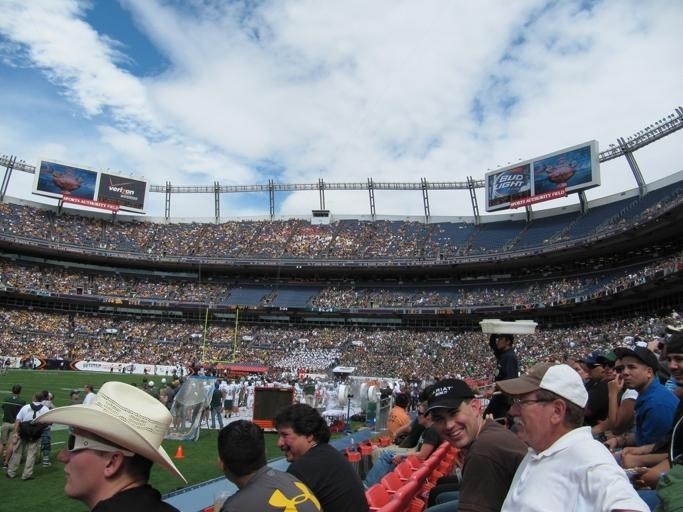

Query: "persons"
<box><xmin>0</xmin><ymin>185</ymin><xmax>683</xmax><ymax>512</ymax></box>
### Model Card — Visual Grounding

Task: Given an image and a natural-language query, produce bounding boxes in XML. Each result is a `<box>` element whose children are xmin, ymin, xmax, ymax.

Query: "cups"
<box><xmin>213</xmin><ymin>491</ymin><xmax>230</xmax><ymax>512</ymax></box>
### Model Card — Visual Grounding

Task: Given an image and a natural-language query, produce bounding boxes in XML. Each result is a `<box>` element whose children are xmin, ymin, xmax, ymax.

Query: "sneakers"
<box><xmin>2</xmin><ymin>460</ymin><xmax>52</xmax><ymax>481</ymax></box>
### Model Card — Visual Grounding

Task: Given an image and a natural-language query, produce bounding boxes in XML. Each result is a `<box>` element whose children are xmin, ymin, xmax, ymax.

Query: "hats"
<box><xmin>423</xmin><ymin>379</ymin><xmax>474</xmax><ymax>417</ymax></box>
<box><xmin>496</xmin><ymin>360</ymin><xmax>589</xmax><ymax>408</ymax></box>
<box><xmin>30</xmin><ymin>381</ymin><xmax>188</xmax><ymax>485</ymax></box>
<box><xmin>667</xmin><ymin>332</ymin><xmax>683</xmax><ymax>354</ymax></box>
<box><xmin>575</xmin><ymin>345</ymin><xmax>658</xmax><ymax>369</ymax></box>
<box><xmin>70</xmin><ymin>389</ymin><xmax>80</xmax><ymax>396</ymax></box>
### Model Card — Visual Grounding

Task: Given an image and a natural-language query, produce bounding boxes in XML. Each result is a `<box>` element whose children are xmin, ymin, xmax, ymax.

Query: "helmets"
<box><xmin>148</xmin><ymin>378</ymin><xmax>166</xmax><ymax>387</ymax></box>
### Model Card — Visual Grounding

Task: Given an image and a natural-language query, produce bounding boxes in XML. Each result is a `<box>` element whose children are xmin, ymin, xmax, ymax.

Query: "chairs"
<box><xmin>198</xmin><ymin>433</ymin><xmax>459</xmax><ymax>512</ymax></box>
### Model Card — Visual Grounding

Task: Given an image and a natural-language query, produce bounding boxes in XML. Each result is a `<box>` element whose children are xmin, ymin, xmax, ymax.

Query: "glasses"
<box><xmin>418</xmin><ymin>410</ymin><xmax>424</xmax><ymax>416</ymax></box>
<box><xmin>67</xmin><ymin>430</ymin><xmax>135</xmax><ymax>457</ymax></box>
<box><xmin>613</xmin><ymin>366</ymin><xmax>623</xmax><ymax>373</ymax></box>
<box><xmin>507</xmin><ymin>396</ymin><xmax>538</xmax><ymax>408</ymax></box>
